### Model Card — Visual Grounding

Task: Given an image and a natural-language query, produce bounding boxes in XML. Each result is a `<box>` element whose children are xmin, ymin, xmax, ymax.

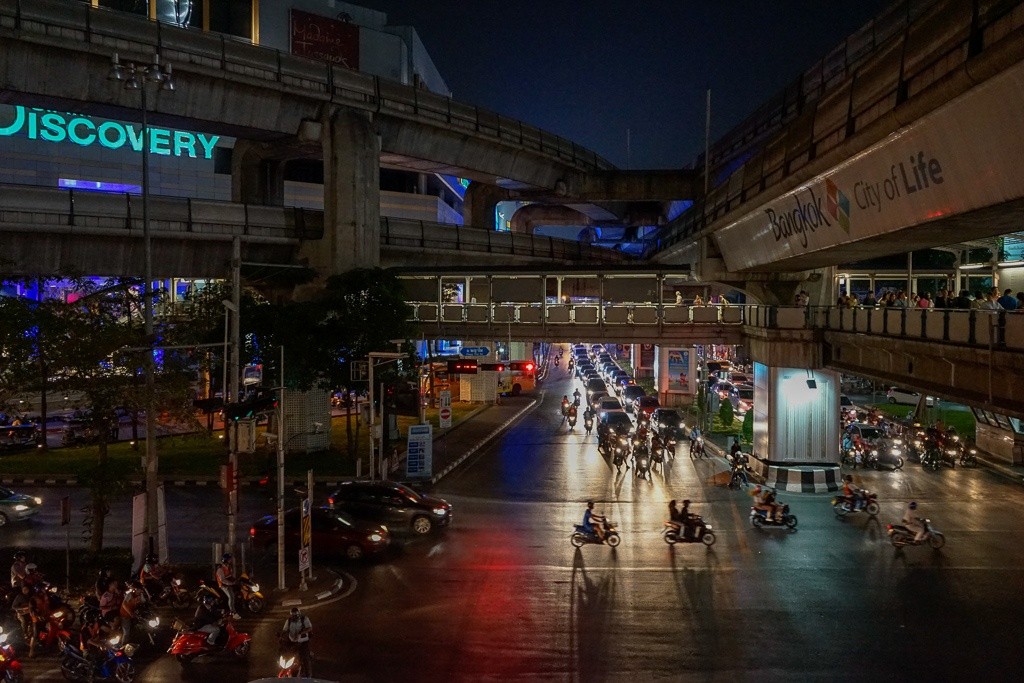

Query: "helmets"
<box><xmin>908</xmin><ymin>502</ymin><xmax>917</xmax><ymax>510</ymax></box>
<box><xmin>0</xmin><ymin>553</ymin><xmax>300</xmax><ymax>623</ymax></box>
<box><xmin>557</xmin><ymin>389</ymin><xmax>972</xmax><ymax>459</ymax></box>
<box><xmin>755</xmin><ymin>485</ymin><xmax>762</xmax><ymax>492</ymax></box>
<box><xmin>771</xmin><ymin>488</ymin><xmax>777</xmax><ymax>495</ymax></box>
<box><xmin>587</xmin><ymin>502</ymin><xmax>593</xmax><ymax>507</ymax></box>
<box><xmin>845</xmin><ymin>475</ymin><xmax>853</xmax><ymax>482</ymax></box>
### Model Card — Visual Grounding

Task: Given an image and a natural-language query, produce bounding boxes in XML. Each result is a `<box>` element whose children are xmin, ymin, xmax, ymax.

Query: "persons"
<box><xmin>690</xmin><ymin>427</ymin><xmax>707</xmax><ymax>457</ymax></box>
<box><xmin>902</xmin><ymin>502</ymin><xmax>928</xmax><ymax>540</ymax></box>
<box><xmin>332</xmin><ymin>388</ymin><xmax>352</xmax><ymax>408</ymax></box>
<box><xmin>749</xmin><ymin>486</ymin><xmax>774</xmax><ymax>521</ymax></box>
<box><xmin>765</xmin><ymin>488</ymin><xmax>785</xmax><ymax>523</ymax></box>
<box><xmin>843</xmin><ymin>476</ymin><xmax>869</xmax><ymax>512</ymax></box>
<box><xmin>840</xmin><ymin>405</ymin><xmax>968</xmax><ymax>467</ymax></box>
<box><xmin>583</xmin><ymin>502</ymin><xmax>606</xmax><ymax>540</ymax></box>
<box><xmin>728</xmin><ymin>451</ymin><xmax>749</xmax><ymax>488</ymax></box>
<box><xmin>730</xmin><ymin>440</ymin><xmax>751</xmax><ymax>472</ymax></box>
<box><xmin>280</xmin><ymin>607</ymin><xmax>312</xmax><ymax>679</ymax></box>
<box><xmin>554</xmin><ymin>345</ymin><xmax>574</xmax><ymax>364</ymax></box>
<box><xmin>0</xmin><ymin>405</ymin><xmax>120</xmax><ymax>445</ymax></box>
<box><xmin>0</xmin><ymin>552</ymin><xmax>241</xmax><ymax>683</ymax></box>
<box><xmin>794</xmin><ymin>286</ymin><xmax>1024</xmax><ymax>311</ymax></box>
<box><xmin>669</xmin><ymin>500</ymin><xmax>687</xmax><ymax>539</ymax></box>
<box><xmin>681</xmin><ymin>500</ymin><xmax>698</xmax><ymax>539</ymax></box>
<box><xmin>676</xmin><ymin>291</ymin><xmax>730</xmax><ymax>321</ymax></box>
<box><xmin>562</xmin><ymin>388</ymin><xmax>676</xmax><ymax>479</ymax></box>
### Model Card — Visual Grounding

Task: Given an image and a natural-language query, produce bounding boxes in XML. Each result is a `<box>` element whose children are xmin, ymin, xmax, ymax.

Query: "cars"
<box><xmin>572</xmin><ymin>342</ymin><xmax>685</xmax><ymax>436</ymax></box>
<box><xmin>247</xmin><ymin>506</ymin><xmax>390</xmax><ymax>561</ymax></box>
<box><xmin>1</xmin><ymin>486</ymin><xmax>43</xmax><ymax>526</ymax></box>
<box><xmin>887</xmin><ymin>386</ymin><xmax>940</xmax><ymax>407</ymax></box>
<box><xmin>697</xmin><ymin>357</ymin><xmax>754</xmax><ymax>416</ymax></box>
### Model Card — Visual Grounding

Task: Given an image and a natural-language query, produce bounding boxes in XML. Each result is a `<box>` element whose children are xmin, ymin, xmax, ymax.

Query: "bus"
<box><xmin>420</xmin><ymin>357</ymin><xmax>538</xmax><ymax>398</ymax></box>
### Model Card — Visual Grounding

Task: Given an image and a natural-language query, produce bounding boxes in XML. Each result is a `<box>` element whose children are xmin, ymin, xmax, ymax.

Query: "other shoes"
<box><xmin>765</xmin><ymin>518</ymin><xmax>773</xmax><ymax>522</ymax></box>
<box><xmin>601</xmin><ymin>534</ymin><xmax>606</xmax><ymax>540</ymax></box>
<box><xmin>775</xmin><ymin>517</ymin><xmax>782</xmax><ymax>521</ymax></box>
<box><xmin>689</xmin><ymin>535</ymin><xmax>697</xmax><ymax>540</ymax></box>
<box><xmin>5</xmin><ymin>627</ymin><xmax>312</xmax><ymax>679</ymax></box>
<box><xmin>853</xmin><ymin>508</ymin><xmax>864</xmax><ymax>512</ymax></box>
<box><xmin>679</xmin><ymin>534</ymin><xmax>687</xmax><ymax>540</ymax></box>
<box><xmin>914</xmin><ymin>537</ymin><xmax>924</xmax><ymax>541</ymax></box>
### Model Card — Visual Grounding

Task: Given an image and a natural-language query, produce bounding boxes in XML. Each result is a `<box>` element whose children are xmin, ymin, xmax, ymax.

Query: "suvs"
<box><xmin>329</xmin><ymin>479</ymin><xmax>455</xmax><ymax>537</ymax></box>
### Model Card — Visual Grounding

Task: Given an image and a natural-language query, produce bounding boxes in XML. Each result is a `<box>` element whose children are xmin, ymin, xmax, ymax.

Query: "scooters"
<box><xmin>568</xmin><ymin>362</ymin><xmax>574</xmax><ymax>372</ymax></box>
<box><xmin>568</xmin><ymin>413</ymin><xmax>578</xmax><ymax>429</ymax></box>
<box><xmin>6</xmin><ymin>407</ymin><xmax>123</xmax><ymax>446</ymax></box>
<box><xmin>839</xmin><ymin>394</ymin><xmax>977</xmax><ymax>472</ymax></box>
<box><xmin>725</xmin><ymin>453</ymin><xmax>751</xmax><ymax>489</ymax></box>
<box><xmin>750</xmin><ymin>502</ymin><xmax>798</xmax><ymax>528</ymax></box>
<box><xmin>888</xmin><ymin>518</ymin><xmax>946</xmax><ymax>554</ymax></box>
<box><xmin>832</xmin><ymin>488</ymin><xmax>881</xmax><ymax>517</ymax></box>
<box><xmin>561</xmin><ymin>402</ymin><xmax>572</xmax><ymax>418</ymax></box>
<box><xmin>554</xmin><ymin>359</ymin><xmax>560</xmax><ymax>366</ymax></box>
<box><xmin>0</xmin><ymin>564</ymin><xmax>314</xmax><ymax>683</ymax></box>
<box><xmin>559</xmin><ymin>349</ymin><xmax>563</xmax><ymax>356</ymax></box>
<box><xmin>330</xmin><ymin>396</ymin><xmax>356</xmax><ymax>409</ymax></box>
<box><xmin>661</xmin><ymin>515</ymin><xmax>717</xmax><ymax>546</ymax></box>
<box><xmin>571</xmin><ymin>512</ymin><xmax>621</xmax><ymax>547</ymax></box>
<box><xmin>600</xmin><ymin>420</ymin><xmax>705</xmax><ymax>478</ymax></box>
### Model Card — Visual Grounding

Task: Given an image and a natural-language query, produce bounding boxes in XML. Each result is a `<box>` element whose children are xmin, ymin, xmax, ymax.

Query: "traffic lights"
<box><xmin>226</xmin><ymin>394</ymin><xmax>281</xmax><ymax>419</ymax></box>
<box><xmin>510</xmin><ymin>362</ymin><xmax>536</xmax><ymax>371</ymax></box>
<box><xmin>481</xmin><ymin>363</ymin><xmax>505</xmax><ymax>371</ymax></box>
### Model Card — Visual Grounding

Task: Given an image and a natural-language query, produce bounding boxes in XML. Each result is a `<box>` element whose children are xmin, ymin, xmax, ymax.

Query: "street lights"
<box><xmin>108</xmin><ymin>50</ymin><xmax>176</xmax><ymax>561</ymax></box>
